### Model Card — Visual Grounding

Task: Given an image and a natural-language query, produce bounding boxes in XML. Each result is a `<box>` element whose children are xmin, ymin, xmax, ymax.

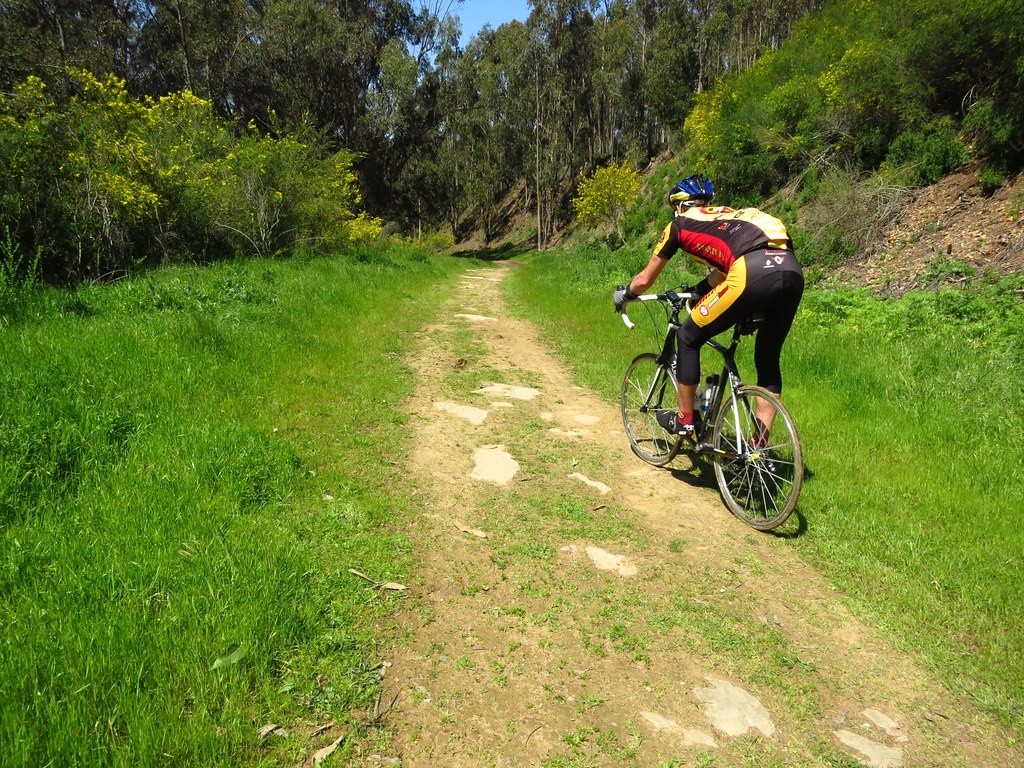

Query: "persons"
<box><xmin>612</xmin><ymin>173</ymin><xmax>804</xmax><ymax>452</ymax></box>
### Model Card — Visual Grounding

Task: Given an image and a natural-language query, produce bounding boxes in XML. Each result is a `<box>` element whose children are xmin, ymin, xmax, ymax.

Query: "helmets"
<box><xmin>668</xmin><ymin>174</ymin><xmax>714</xmax><ymax>203</ymax></box>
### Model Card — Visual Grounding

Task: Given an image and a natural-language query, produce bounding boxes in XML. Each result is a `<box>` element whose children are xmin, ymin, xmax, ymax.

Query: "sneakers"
<box><xmin>656</xmin><ymin>409</ymin><xmax>694</xmax><ymax>440</ymax></box>
<box><xmin>727</xmin><ymin>446</ymin><xmax>770</xmax><ymax>463</ymax></box>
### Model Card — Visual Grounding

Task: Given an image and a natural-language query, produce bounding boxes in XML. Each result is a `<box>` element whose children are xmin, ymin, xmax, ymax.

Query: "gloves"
<box><xmin>613</xmin><ymin>289</ymin><xmax>627</xmax><ymax>314</ymax></box>
<box><xmin>680</xmin><ymin>284</ymin><xmax>698</xmax><ymax>309</ymax></box>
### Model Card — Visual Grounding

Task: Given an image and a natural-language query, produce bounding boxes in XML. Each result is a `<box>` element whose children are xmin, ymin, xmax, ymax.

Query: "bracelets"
<box><xmin>623</xmin><ymin>282</ymin><xmax>638</xmax><ymax>300</ymax></box>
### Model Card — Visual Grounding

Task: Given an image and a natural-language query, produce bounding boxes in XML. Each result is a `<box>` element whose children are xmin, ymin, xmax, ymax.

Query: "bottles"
<box><xmin>700</xmin><ymin>376</ymin><xmax>713</xmax><ymax>417</ymax></box>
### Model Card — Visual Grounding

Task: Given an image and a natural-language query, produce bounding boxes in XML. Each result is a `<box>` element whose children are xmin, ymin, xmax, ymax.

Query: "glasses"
<box><xmin>672</xmin><ymin>207</ymin><xmax>679</xmax><ymax>218</ymax></box>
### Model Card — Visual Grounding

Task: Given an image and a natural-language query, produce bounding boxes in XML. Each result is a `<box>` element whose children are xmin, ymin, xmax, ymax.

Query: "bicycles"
<box><xmin>615</xmin><ymin>283</ymin><xmax>803</xmax><ymax>531</ymax></box>
